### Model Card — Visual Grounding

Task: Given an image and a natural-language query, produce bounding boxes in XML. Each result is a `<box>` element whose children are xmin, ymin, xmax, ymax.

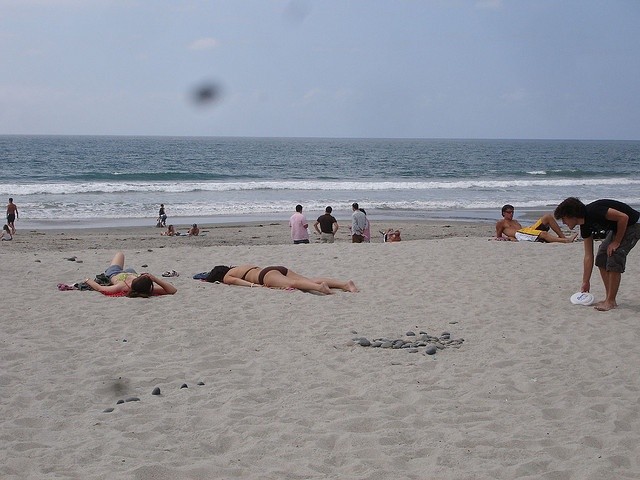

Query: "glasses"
<box><xmin>503</xmin><ymin>211</ymin><xmax>514</xmax><ymax>214</ymax></box>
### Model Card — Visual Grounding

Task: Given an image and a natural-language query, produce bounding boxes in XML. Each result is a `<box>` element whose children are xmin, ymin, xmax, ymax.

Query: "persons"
<box><xmin>188</xmin><ymin>223</ymin><xmax>199</xmax><ymax>236</ymax></box>
<box><xmin>0</xmin><ymin>225</ymin><xmax>13</xmax><ymax>241</ymax></box>
<box><xmin>351</xmin><ymin>202</ymin><xmax>369</xmax><ymax>242</ymax></box>
<box><xmin>495</xmin><ymin>204</ymin><xmax>579</xmax><ymax>244</ymax></box>
<box><xmin>201</xmin><ymin>265</ymin><xmax>358</xmax><ymax>294</ymax></box>
<box><xmin>160</xmin><ymin>225</ymin><xmax>175</xmax><ymax>236</ymax></box>
<box><xmin>156</xmin><ymin>204</ymin><xmax>167</xmax><ymax>228</ymax></box>
<box><xmin>6</xmin><ymin>197</ymin><xmax>19</xmax><ymax>234</ymax></box>
<box><xmin>84</xmin><ymin>251</ymin><xmax>178</xmax><ymax>299</ymax></box>
<box><xmin>382</xmin><ymin>227</ymin><xmax>402</xmax><ymax>242</ymax></box>
<box><xmin>314</xmin><ymin>206</ymin><xmax>338</xmax><ymax>243</ymax></box>
<box><xmin>288</xmin><ymin>205</ymin><xmax>309</xmax><ymax>244</ymax></box>
<box><xmin>554</xmin><ymin>197</ymin><xmax>640</xmax><ymax>312</ymax></box>
<box><xmin>359</xmin><ymin>208</ymin><xmax>371</xmax><ymax>242</ymax></box>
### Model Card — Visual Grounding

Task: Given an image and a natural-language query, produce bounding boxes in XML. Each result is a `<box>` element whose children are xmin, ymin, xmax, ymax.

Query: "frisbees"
<box><xmin>571</xmin><ymin>293</ymin><xmax>594</xmax><ymax>306</ymax></box>
